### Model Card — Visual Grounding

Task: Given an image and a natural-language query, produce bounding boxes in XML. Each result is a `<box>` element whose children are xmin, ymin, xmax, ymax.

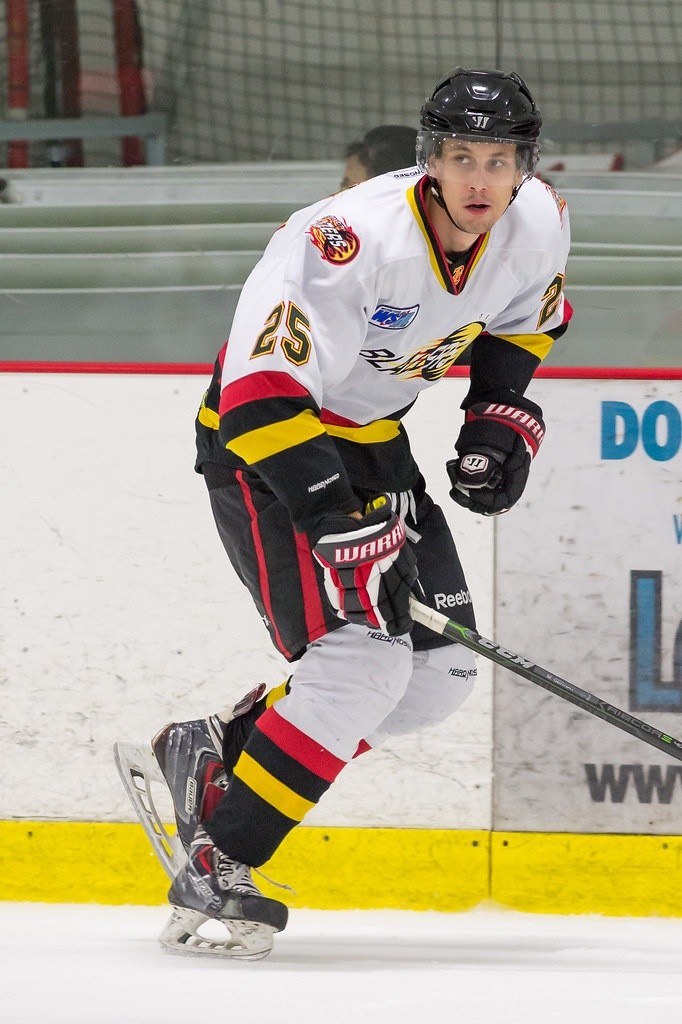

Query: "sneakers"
<box><xmin>113</xmin><ymin>683</ymin><xmax>267</xmax><ymax>881</ymax></box>
<box><xmin>157</xmin><ymin>824</ymin><xmax>288</xmax><ymax>962</ymax></box>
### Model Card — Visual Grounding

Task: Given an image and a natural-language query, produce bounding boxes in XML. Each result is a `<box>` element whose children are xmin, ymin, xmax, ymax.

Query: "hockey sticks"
<box><xmin>406</xmin><ymin>593</ymin><xmax>682</xmax><ymax>768</ymax></box>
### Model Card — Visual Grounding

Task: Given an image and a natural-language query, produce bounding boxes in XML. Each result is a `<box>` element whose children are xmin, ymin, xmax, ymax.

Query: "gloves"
<box><xmin>446</xmin><ymin>381</ymin><xmax>546</xmax><ymax>516</ymax></box>
<box><xmin>309</xmin><ymin>493</ymin><xmax>428</xmax><ymax>637</ymax></box>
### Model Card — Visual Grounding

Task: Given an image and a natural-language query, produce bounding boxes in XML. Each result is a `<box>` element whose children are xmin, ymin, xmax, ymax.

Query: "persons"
<box><xmin>112</xmin><ymin>67</ymin><xmax>571</xmax><ymax>962</ymax></box>
<box><xmin>341</xmin><ymin>125</ymin><xmax>421</xmax><ymax>191</ymax></box>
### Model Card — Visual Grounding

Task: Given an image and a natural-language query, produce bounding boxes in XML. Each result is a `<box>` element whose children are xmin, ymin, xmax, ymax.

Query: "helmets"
<box><xmin>418</xmin><ymin>65</ymin><xmax>543</xmax><ymax>172</ymax></box>
